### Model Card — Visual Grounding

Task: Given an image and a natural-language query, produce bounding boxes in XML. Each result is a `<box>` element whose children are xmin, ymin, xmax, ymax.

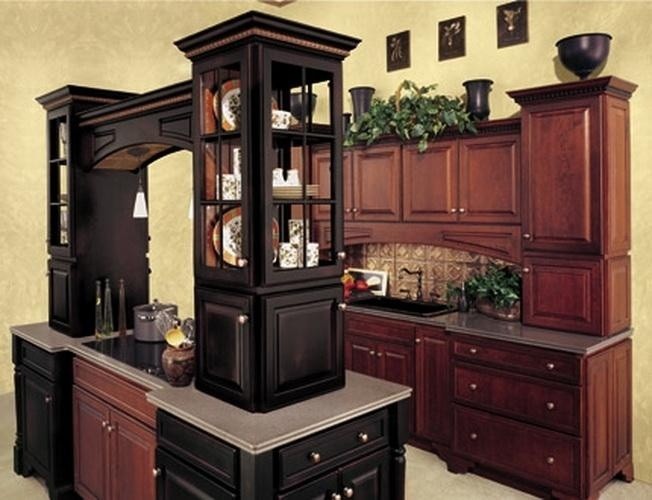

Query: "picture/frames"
<box><xmin>385</xmin><ymin>29</ymin><xmax>411</xmax><ymax>73</ymax></box>
<box><xmin>497</xmin><ymin>0</ymin><xmax>529</xmax><ymax>50</ymax></box>
<box><xmin>437</xmin><ymin>15</ymin><xmax>466</xmax><ymax>63</ymax></box>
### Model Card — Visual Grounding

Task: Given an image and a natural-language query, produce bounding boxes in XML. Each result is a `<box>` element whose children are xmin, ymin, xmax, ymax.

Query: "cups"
<box><xmin>278</xmin><ymin>242</ymin><xmax>300</xmax><ymax>270</ymax></box>
<box><xmin>273</xmin><ymin>169</ymin><xmax>288</xmax><ymax>187</ymax></box>
<box><xmin>300</xmin><ymin>244</ymin><xmax>320</xmax><ymax>268</ymax></box>
<box><xmin>286</xmin><ymin>170</ymin><xmax>302</xmax><ymax>187</ymax></box>
<box><xmin>215</xmin><ymin>175</ymin><xmax>238</xmax><ymax>200</ymax></box>
<box><xmin>272</xmin><ymin>110</ymin><xmax>292</xmax><ymax>130</ymax></box>
<box><xmin>289</xmin><ymin>220</ymin><xmax>310</xmax><ymax>246</ymax></box>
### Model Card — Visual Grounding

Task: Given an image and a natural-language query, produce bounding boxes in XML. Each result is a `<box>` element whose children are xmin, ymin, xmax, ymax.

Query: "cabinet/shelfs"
<box><xmin>414</xmin><ymin>324</ymin><xmax>450</xmax><ymax>459</ymax></box>
<box><xmin>285</xmin><ymin>119</ymin><xmax>333</xmax><ymax>225</ymax></box>
<box><xmin>507</xmin><ymin>74</ymin><xmax>639</xmax><ymax>259</ymax></box>
<box><xmin>397</xmin><ymin>131</ymin><xmax>519</xmax><ymax>227</ymax></box>
<box><xmin>32</xmin><ymin>9</ymin><xmax>364</xmax><ymax>413</ymax></box>
<box><xmin>448</xmin><ymin>331</ymin><xmax>635</xmax><ymax>499</ymax></box>
<box><xmin>148</xmin><ymin>367</ymin><xmax>415</xmax><ymax>499</ymax></box>
<box><xmin>343</xmin><ymin>311</ymin><xmax>417</xmax><ymax>448</ymax></box>
<box><xmin>68</xmin><ymin>328</ymin><xmax>169</xmax><ymax>499</ymax></box>
<box><xmin>311</xmin><ymin>143</ymin><xmax>400</xmax><ymax>225</ymax></box>
<box><xmin>520</xmin><ymin>251</ymin><xmax>633</xmax><ymax>338</ymax></box>
<box><xmin>11</xmin><ymin>322</ymin><xmax>144</xmax><ymax>499</ymax></box>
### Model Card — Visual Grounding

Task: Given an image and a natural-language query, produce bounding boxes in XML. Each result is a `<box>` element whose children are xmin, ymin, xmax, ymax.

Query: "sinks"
<box><xmin>349</xmin><ymin>296</ymin><xmax>459</xmax><ymax>315</ymax></box>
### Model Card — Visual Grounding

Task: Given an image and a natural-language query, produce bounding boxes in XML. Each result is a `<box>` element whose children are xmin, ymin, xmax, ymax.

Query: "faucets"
<box><xmin>398</xmin><ymin>269</ymin><xmax>425</xmax><ymax>304</ymax></box>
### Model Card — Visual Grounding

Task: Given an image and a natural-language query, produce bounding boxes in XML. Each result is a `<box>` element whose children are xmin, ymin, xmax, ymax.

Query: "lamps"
<box><xmin>124</xmin><ymin>146</ymin><xmax>154</xmax><ymax>219</ymax></box>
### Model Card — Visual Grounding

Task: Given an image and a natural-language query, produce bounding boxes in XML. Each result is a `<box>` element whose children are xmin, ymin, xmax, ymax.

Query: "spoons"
<box><xmin>165</xmin><ymin>330</ymin><xmax>186</xmax><ymax>349</ymax></box>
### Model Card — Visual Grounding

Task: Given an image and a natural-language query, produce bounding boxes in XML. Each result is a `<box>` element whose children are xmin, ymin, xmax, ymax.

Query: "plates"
<box><xmin>272</xmin><ymin>184</ymin><xmax>321</xmax><ymax>200</ymax></box>
<box><xmin>212</xmin><ymin>79</ymin><xmax>279</xmax><ymax>131</ymax></box>
<box><xmin>212</xmin><ymin>206</ymin><xmax>280</xmax><ymax>266</ymax></box>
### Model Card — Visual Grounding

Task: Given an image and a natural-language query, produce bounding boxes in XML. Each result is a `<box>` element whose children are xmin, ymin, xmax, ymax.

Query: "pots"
<box><xmin>132</xmin><ymin>299</ymin><xmax>177</xmax><ymax>342</ymax></box>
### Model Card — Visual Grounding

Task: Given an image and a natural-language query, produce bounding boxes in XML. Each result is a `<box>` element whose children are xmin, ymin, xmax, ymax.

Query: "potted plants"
<box><xmin>464</xmin><ymin>262</ymin><xmax>521</xmax><ymax>322</ymax></box>
